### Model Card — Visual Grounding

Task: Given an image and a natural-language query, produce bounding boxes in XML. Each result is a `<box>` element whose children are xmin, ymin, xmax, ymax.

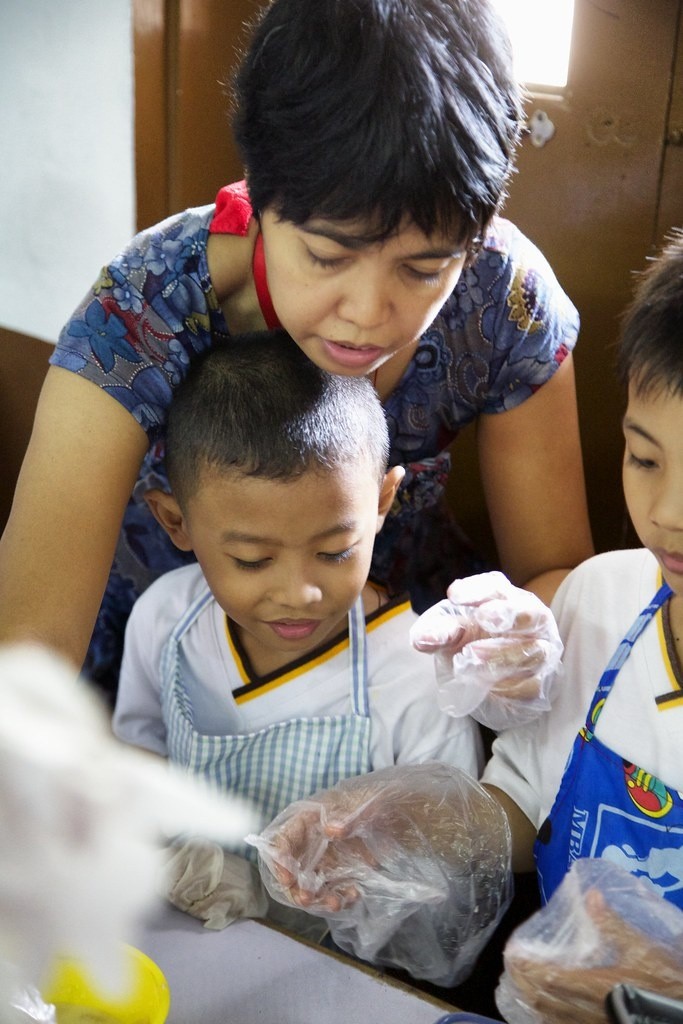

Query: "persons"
<box><xmin>0</xmin><ymin>0</ymin><xmax>594</xmax><ymax>938</ymax></box>
<box><xmin>243</xmin><ymin>237</ymin><xmax>683</xmax><ymax>1024</ymax></box>
<box><xmin>109</xmin><ymin>331</ymin><xmax>484</xmax><ymax>930</ymax></box>
